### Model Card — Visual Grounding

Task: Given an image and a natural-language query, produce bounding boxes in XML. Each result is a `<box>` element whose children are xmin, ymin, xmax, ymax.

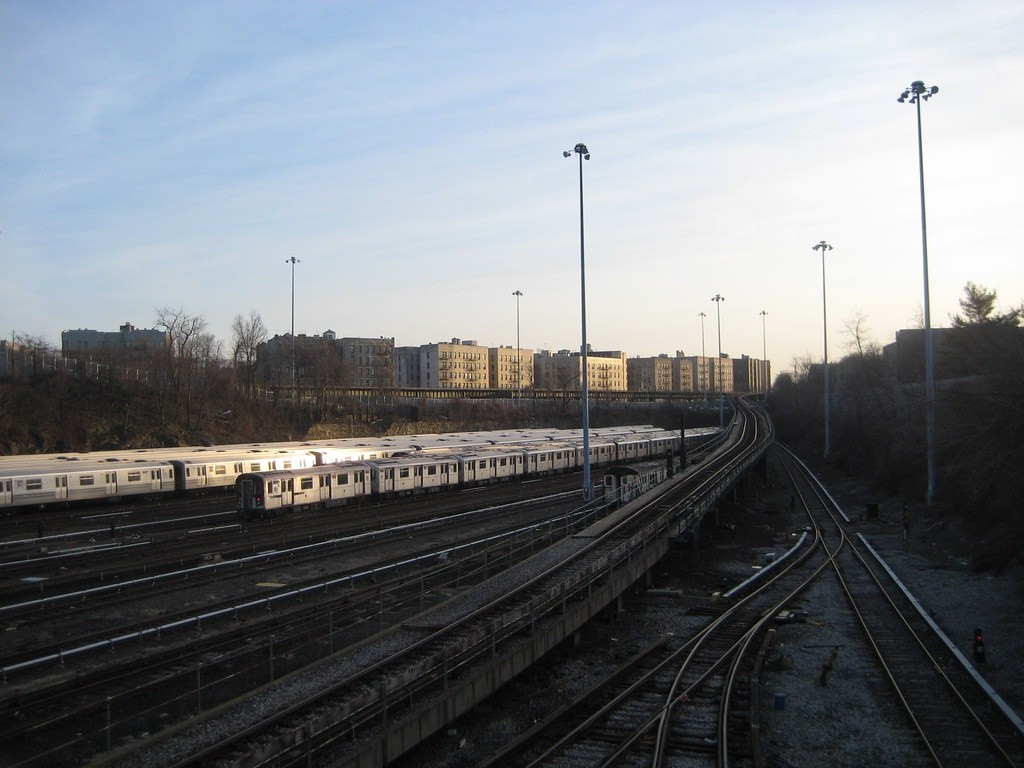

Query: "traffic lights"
<box><xmin>971</xmin><ymin>629</ymin><xmax>986</xmax><ymax>662</ymax></box>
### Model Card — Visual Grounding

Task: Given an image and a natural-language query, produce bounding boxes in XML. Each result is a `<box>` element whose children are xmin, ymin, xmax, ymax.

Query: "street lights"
<box><xmin>512</xmin><ymin>289</ymin><xmax>524</xmax><ymax>413</ymax></box>
<box><xmin>285</xmin><ymin>256</ymin><xmax>301</xmax><ymax>420</ymax></box>
<box><xmin>561</xmin><ymin>142</ymin><xmax>599</xmax><ymax>502</ymax></box>
<box><xmin>710</xmin><ymin>293</ymin><xmax>727</xmax><ymax>431</ymax></box>
<box><xmin>896</xmin><ymin>77</ymin><xmax>942</xmax><ymax>521</ymax></box>
<box><xmin>698</xmin><ymin>311</ymin><xmax>708</xmax><ymax>428</ymax></box>
<box><xmin>756</xmin><ymin>310</ymin><xmax>771</xmax><ymax>407</ymax></box>
<box><xmin>810</xmin><ymin>241</ymin><xmax>836</xmax><ymax>463</ymax></box>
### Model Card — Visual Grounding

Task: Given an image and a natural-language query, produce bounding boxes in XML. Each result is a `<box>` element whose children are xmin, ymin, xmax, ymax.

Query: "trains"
<box><xmin>0</xmin><ymin>417</ymin><xmax>723</xmax><ymax>521</ymax></box>
